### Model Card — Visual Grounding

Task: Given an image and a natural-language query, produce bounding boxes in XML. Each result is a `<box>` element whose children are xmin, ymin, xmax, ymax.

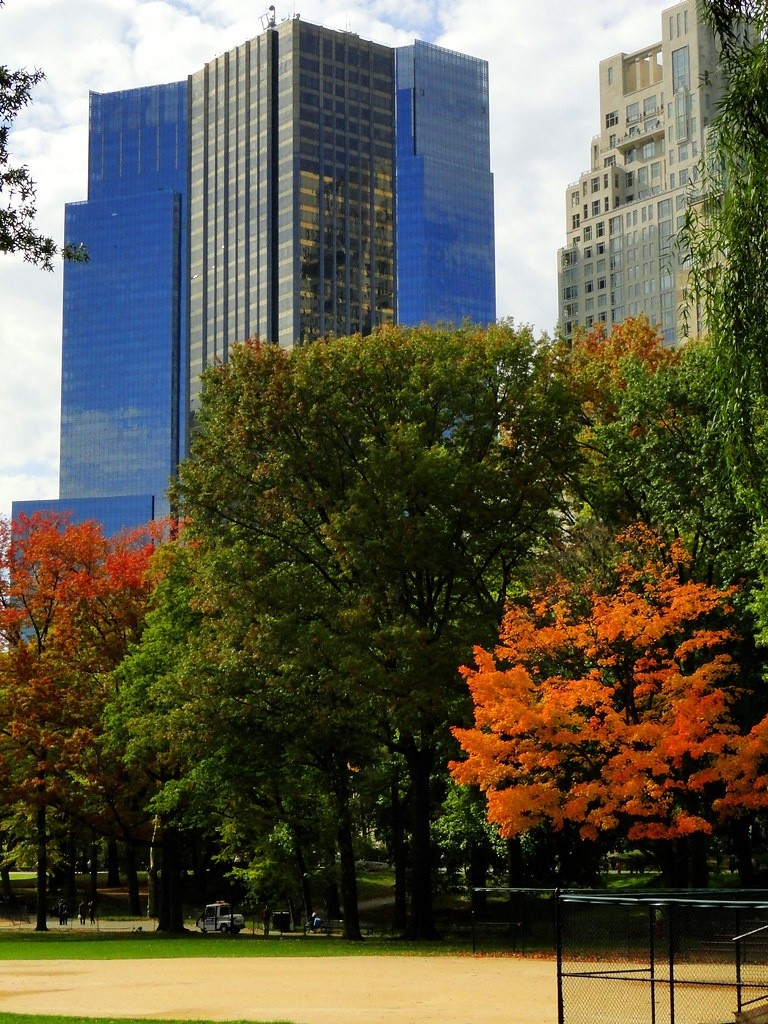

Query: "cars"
<box><xmin>197</xmin><ymin>902</ymin><xmax>245</xmax><ymax>936</ymax></box>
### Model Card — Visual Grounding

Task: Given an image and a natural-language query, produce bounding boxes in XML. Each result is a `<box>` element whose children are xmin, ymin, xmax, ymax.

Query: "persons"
<box><xmin>603</xmin><ymin>853</ymin><xmax>646</xmax><ymax>873</ymax></box>
<box><xmin>261</xmin><ymin>904</ymin><xmax>274</xmax><ymax>937</ymax></box>
<box><xmin>306</xmin><ymin>913</ymin><xmax>321</xmax><ymax>934</ymax></box>
<box><xmin>58</xmin><ymin>898</ymin><xmax>95</xmax><ymax>926</ymax></box>
<box><xmin>729</xmin><ymin>854</ymin><xmax>736</xmax><ymax>873</ymax></box>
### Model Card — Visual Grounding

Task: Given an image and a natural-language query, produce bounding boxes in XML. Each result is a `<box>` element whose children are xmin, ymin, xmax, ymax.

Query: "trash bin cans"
<box><xmin>272</xmin><ymin>909</ymin><xmax>290</xmax><ymax>932</ymax></box>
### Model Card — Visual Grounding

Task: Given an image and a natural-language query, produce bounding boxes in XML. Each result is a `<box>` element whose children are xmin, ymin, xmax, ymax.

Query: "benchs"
<box><xmin>307</xmin><ymin>919</ymin><xmax>373</xmax><ymax>936</ymax></box>
